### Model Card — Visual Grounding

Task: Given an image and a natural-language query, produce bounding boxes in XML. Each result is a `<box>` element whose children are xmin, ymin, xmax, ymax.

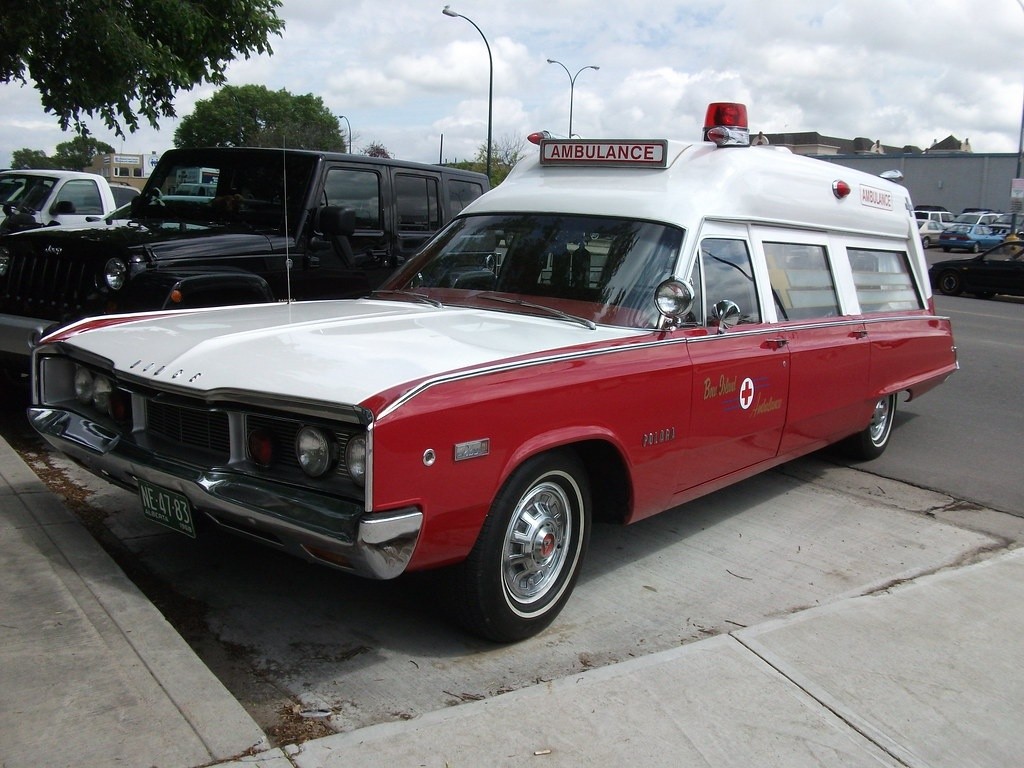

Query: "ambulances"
<box><xmin>26</xmin><ymin>101</ymin><xmax>961</xmax><ymax>650</ymax></box>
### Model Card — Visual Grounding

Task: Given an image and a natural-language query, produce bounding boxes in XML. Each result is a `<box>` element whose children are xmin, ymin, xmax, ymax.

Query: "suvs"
<box><xmin>0</xmin><ymin>179</ymin><xmax>141</xmax><ymax>212</ymax></box>
<box><xmin>0</xmin><ymin>146</ymin><xmax>503</xmax><ymax>375</ymax></box>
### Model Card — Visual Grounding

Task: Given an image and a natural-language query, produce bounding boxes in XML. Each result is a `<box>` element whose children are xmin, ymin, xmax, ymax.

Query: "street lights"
<box><xmin>338</xmin><ymin>116</ymin><xmax>351</xmax><ymax>154</ymax></box>
<box><xmin>442</xmin><ymin>9</ymin><xmax>493</xmax><ymax>186</ymax></box>
<box><xmin>548</xmin><ymin>58</ymin><xmax>600</xmax><ymax>139</ymax></box>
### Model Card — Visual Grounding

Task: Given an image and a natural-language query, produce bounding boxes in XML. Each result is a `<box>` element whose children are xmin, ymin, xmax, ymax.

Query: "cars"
<box><xmin>929</xmin><ymin>240</ymin><xmax>1024</xmax><ymax>296</ymax></box>
<box><xmin>914</xmin><ymin>211</ymin><xmax>957</xmax><ymax>228</ymax></box>
<box><xmin>990</xmin><ymin>213</ymin><xmax>1024</xmax><ymax>239</ymax></box>
<box><xmin>916</xmin><ymin>219</ymin><xmax>947</xmax><ymax>249</ymax></box>
<box><xmin>945</xmin><ymin>211</ymin><xmax>1004</xmax><ymax>230</ymax></box>
<box><xmin>939</xmin><ymin>224</ymin><xmax>1004</xmax><ymax>254</ymax></box>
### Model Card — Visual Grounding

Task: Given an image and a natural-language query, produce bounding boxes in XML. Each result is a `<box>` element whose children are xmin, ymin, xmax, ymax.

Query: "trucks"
<box><xmin>0</xmin><ymin>170</ymin><xmax>207</xmax><ymax>231</ymax></box>
